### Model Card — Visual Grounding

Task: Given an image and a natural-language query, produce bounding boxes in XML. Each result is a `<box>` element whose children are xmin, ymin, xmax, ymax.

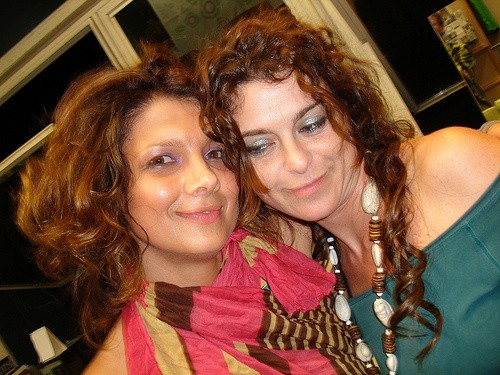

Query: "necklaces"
<box><xmin>324</xmin><ymin>171</ymin><xmax>400</xmax><ymax>375</ymax></box>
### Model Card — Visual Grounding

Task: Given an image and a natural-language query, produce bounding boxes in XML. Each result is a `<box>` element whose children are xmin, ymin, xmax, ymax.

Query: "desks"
<box><xmin>33</xmin><ymin>329</ymin><xmax>96</xmax><ymax>375</ymax></box>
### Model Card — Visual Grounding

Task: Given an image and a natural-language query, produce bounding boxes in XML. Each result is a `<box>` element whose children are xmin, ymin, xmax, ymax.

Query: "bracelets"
<box><xmin>479</xmin><ymin>119</ymin><xmax>499</xmax><ymax>134</ymax></box>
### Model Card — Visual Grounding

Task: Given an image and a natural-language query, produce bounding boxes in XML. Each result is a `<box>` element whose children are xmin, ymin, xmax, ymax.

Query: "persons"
<box><xmin>18</xmin><ymin>45</ymin><xmax>500</xmax><ymax>375</ymax></box>
<box><xmin>191</xmin><ymin>14</ymin><xmax>499</xmax><ymax>375</ymax></box>
